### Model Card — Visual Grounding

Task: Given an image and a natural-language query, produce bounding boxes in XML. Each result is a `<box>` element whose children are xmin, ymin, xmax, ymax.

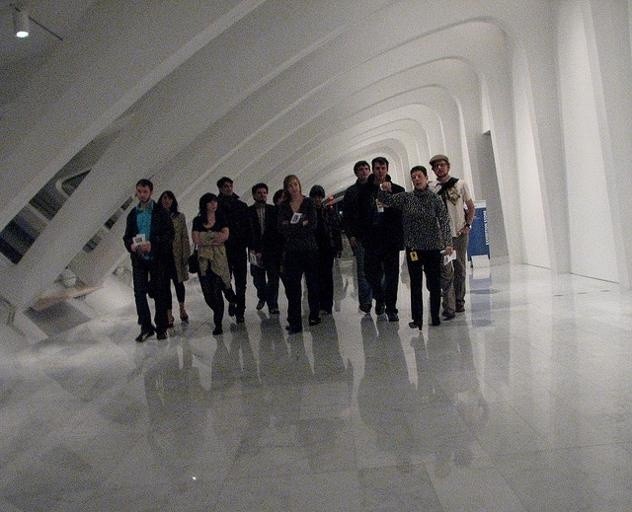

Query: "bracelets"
<box><xmin>465</xmin><ymin>221</ymin><xmax>473</xmax><ymax>230</ymax></box>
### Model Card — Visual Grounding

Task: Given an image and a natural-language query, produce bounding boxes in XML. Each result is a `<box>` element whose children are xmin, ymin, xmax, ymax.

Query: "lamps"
<box><xmin>12</xmin><ymin>11</ymin><xmax>31</xmax><ymax>39</ymax></box>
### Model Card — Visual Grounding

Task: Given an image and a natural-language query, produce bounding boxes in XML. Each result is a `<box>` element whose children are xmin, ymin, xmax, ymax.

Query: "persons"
<box><xmin>355</xmin><ymin>157</ymin><xmax>407</xmax><ymax>320</ymax></box>
<box><xmin>245</xmin><ymin>172</ymin><xmax>346</xmax><ymax>335</ymax></box>
<box><xmin>191</xmin><ymin>193</ymin><xmax>231</xmax><ymax>335</ymax></box>
<box><xmin>159</xmin><ymin>189</ymin><xmax>191</xmax><ymax>326</ymax></box>
<box><xmin>343</xmin><ymin>161</ymin><xmax>386</xmax><ymax>315</ymax></box>
<box><xmin>121</xmin><ymin>178</ymin><xmax>175</xmax><ymax>343</ymax></box>
<box><xmin>214</xmin><ymin>177</ymin><xmax>252</xmax><ymax>324</ymax></box>
<box><xmin>375</xmin><ymin>166</ymin><xmax>453</xmax><ymax>330</ymax></box>
<box><xmin>425</xmin><ymin>156</ymin><xmax>476</xmax><ymax>319</ymax></box>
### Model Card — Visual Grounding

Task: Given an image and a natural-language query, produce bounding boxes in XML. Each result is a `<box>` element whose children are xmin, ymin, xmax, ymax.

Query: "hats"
<box><xmin>429</xmin><ymin>155</ymin><xmax>448</xmax><ymax>165</ymax></box>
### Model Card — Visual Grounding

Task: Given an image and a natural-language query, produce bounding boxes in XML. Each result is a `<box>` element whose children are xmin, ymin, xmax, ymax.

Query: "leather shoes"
<box><xmin>213</xmin><ymin>297</ymin><xmax>332</xmax><ymax>334</ymax></box>
<box><xmin>359</xmin><ymin>301</ymin><xmax>465</xmax><ymax>330</ymax></box>
<box><xmin>135</xmin><ymin>308</ymin><xmax>188</xmax><ymax>342</ymax></box>
